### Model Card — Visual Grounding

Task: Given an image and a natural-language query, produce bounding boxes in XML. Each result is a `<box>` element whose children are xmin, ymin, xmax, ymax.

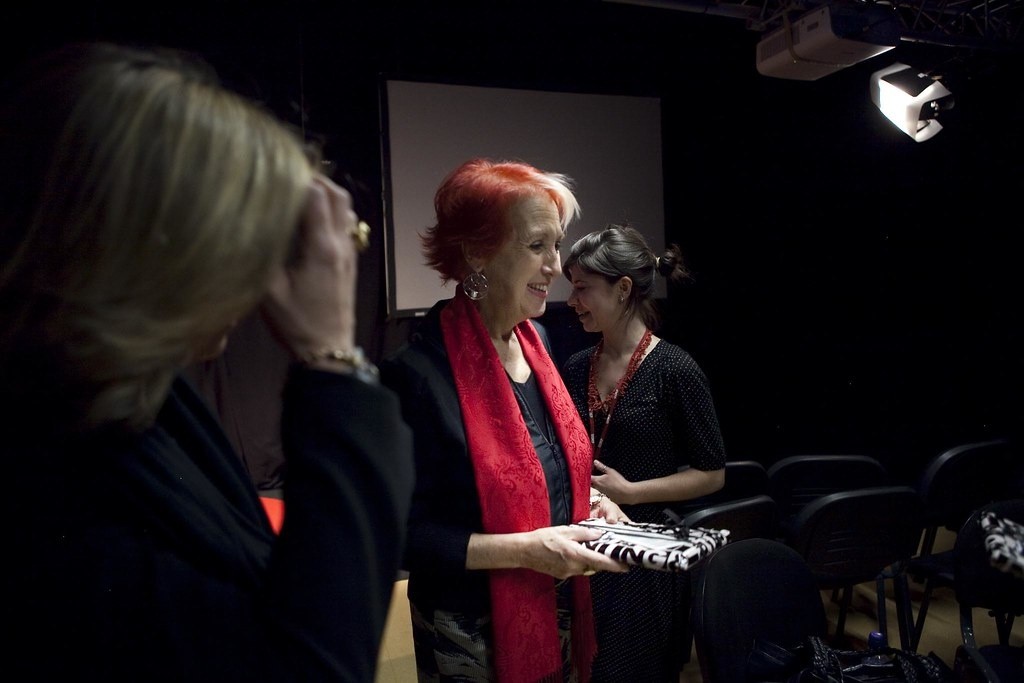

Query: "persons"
<box><xmin>560</xmin><ymin>224</ymin><xmax>726</xmax><ymax>683</ymax></box>
<box><xmin>1</xmin><ymin>45</ymin><xmax>417</xmax><ymax>683</ymax></box>
<box><xmin>376</xmin><ymin>157</ymin><xmax>636</xmax><ymax>683</ymax></box>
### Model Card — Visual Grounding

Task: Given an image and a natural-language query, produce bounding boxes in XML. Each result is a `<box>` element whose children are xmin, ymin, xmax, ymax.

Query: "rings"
<box><xmin>582</xmin><ymin>567</ymin><xmax>596</xmax><ymax>576</ymax></box>
<box><xmin>350</xmin><ymin>220</ymin><xmax>371</xmax><ymax>249</ymax></box>
<box><xmin>602</xmin><ymin>465</ymin><xmax>606</xmax><ymax>473</ymax></box>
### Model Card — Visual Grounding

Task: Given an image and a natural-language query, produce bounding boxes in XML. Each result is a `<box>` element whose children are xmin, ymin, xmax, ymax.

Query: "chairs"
<box><xmin>676</xmin><ymin>439</ymin><xmax>1024</xmax><ymax>683</ymax></box>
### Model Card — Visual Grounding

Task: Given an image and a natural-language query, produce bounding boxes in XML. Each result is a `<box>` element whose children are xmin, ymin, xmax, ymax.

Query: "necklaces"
<box><xmin>587</xmin><ymin>330</ymin><xmax>652</xmax><ymax>461</ymax></box>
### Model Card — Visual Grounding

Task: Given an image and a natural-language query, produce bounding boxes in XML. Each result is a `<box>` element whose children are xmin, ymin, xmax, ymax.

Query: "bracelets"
<box><xmin>305</xmin><ymin>345</ymin><xmax>379</xmax><ymax>375</ymax></box>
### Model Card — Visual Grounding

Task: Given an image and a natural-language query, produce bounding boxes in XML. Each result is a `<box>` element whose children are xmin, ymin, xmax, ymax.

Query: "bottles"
<box><xmin>862</xmin><ymin>632</ymin><xmax>884</xmax><ymax>671</ymax></box>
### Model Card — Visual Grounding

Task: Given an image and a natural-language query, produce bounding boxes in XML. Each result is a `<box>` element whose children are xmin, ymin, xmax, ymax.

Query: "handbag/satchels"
<box><xmin>739</xmin><ymin>637</ymin><xmax>945</xmax><ymax>683</ymax></box>
<box><xmin>976</xmin><ymin>511</ymin><xmax>1024</xmax><ymax>577</ymax></box>
